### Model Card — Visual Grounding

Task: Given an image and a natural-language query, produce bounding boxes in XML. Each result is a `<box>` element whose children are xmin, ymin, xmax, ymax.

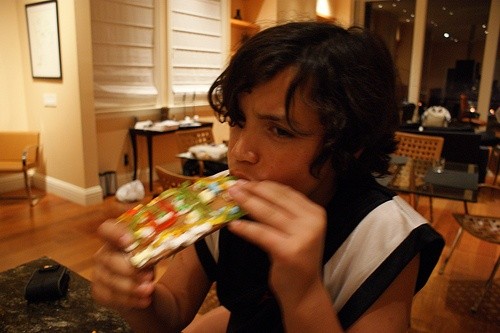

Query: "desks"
<box><xmin>409</xmin><ymin>118</ymin><xmax>475</xmax><ymax>162</ymax></box>
<box><xmin>175</xmin><ymin>141</ymin><xmax>479</xmax><ymax>221</ymax></box>
<box><xmin>127</xmin><ymin>119</ymin><xmax>214</xmax><ymax>192</ymax></box>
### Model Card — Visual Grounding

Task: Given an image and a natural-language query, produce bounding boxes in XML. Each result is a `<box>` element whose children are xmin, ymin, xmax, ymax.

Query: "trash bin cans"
<box><xmin>99</xmin><ymin>171</ymin><xmax>117</xmax><ymax>197</ymax></box>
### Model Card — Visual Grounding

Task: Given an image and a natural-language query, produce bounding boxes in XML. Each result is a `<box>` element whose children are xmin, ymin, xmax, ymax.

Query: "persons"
<box><xmin>421</xmin><ymin>98</ymin><xmax>451</xmax><ymax>128</ymax></box>
<box><xmin>91</xmin><ymin>20</ymin><xmax>446</xmax><ymax>333</ymax></box>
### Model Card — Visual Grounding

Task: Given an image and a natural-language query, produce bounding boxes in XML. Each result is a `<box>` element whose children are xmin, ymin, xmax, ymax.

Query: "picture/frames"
<box><xmin>25</xmin><ymin>0</ymin><xmax>63</xmax><ymax>79</ymax></box>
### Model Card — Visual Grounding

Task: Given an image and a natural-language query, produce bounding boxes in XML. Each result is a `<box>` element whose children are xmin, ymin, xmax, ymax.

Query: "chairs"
<box><xmin>438</xmin><ymin>180</ymin><xmax>500</xmax><ymax>314</ymax></box>
<box><xmin>390</xmin><ymin>132</ymin><xmax>444</xmax><ymax>225</ymax></box>
<box><xmin>155</xmin><ymin>127</ymin><xmax>216</xmax><ymax>191</ymax></box>
<box><xmin>0</xmin><ymin>131</ymin><xmax>43</xmax><ymax>206</ymax></box>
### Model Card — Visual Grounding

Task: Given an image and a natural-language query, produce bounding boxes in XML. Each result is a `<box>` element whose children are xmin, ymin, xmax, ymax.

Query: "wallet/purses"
<box><xmin>23</xmin><ymin>265</ymin><xmax>70</xmax><ymax>304</ymax></box>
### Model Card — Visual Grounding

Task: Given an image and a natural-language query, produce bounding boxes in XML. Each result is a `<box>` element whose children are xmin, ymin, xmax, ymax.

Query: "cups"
<box><xmin>431</xmin><ymin>158</ymin><xmax>445</xmax><ymax>174</ymax></box>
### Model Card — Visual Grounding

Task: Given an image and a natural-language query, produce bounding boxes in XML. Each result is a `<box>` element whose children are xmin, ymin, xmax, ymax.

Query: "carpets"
<box><xmin>0</xmin><ymin>255</ymin><xmax>131</xmax><ymax>333</ymax></box>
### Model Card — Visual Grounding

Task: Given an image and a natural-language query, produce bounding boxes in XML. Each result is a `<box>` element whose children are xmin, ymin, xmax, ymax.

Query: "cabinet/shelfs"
<box><xmin>228</xmin><ymin>0</ymin><xmax>356</xmax><ymax>56</ymax></box>
